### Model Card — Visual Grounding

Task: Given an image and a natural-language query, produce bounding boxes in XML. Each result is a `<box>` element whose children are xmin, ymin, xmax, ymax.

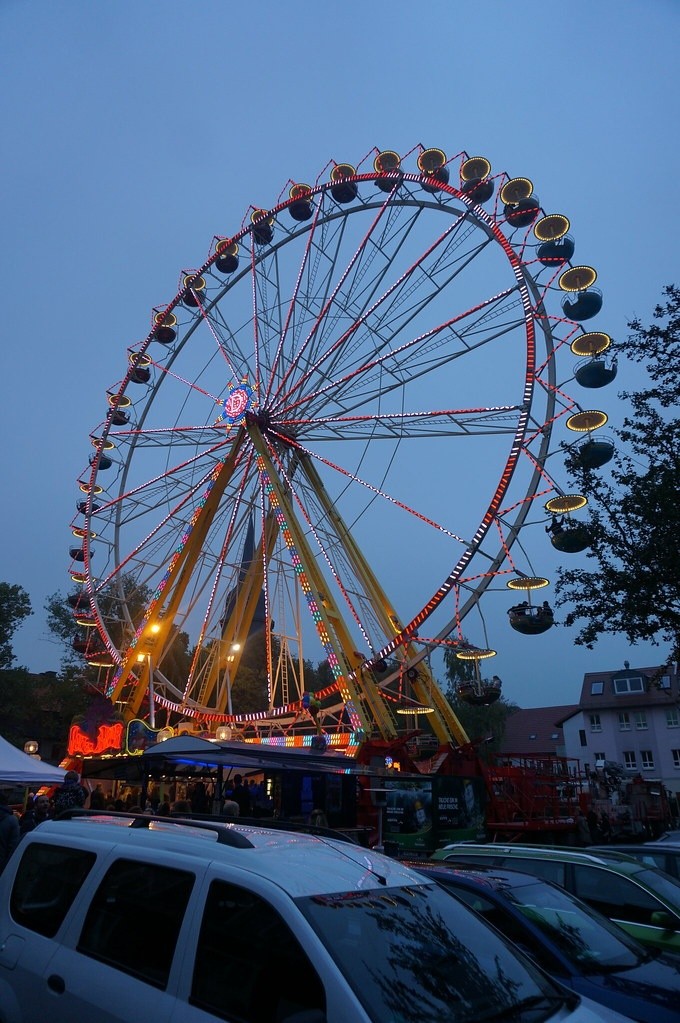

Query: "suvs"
<box><xmin>429</xmin><ymin>838</ymin><xmax>680</xmax><ymax>968</ymax></box>
<box><xmin>0</xmin><ymin>806</ymin><xmax>637</xmax><ymax>1022</ymax></box>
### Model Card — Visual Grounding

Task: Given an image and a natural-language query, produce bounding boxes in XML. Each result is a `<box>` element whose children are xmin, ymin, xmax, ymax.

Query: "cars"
<box><xmin>380</xmin><ymin>852</ymin><xmax>679</xmax><ymax>1023</ymax></box>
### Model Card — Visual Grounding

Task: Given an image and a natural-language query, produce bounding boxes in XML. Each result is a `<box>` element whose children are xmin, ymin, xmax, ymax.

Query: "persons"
<box><xmin>569</xmin><ymin>798</ymin><xmax>680</xmax><ymax>847</ymax></box>
<box><xmin>489</xmin><ymin>676</ymin><xmax>503</xmax><ymax>691</ymax></box>
<box><xmin>0</xmin><ymin>769</ymin><xmax>327</xmax><ymax>865</ymax></box>
<box><xmin>509</xmin><ymin>599</ymin><xmax>554</xmax><ymax>618</ymax></box>
<box><xmin>546</xmin><ymin>513</ymin><xmax>566</xmax><ymax>534</ymax></box>
<box><xmin>145</xmin><ymin>366</ymin><xmax>150</xmax><ymax>373</ymax></box>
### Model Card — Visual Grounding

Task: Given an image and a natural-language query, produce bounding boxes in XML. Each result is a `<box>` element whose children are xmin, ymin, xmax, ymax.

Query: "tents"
<box><xmin>143</xmin><ymin>737</ymin><xmax>356</xmax><ymax>826</ymax></box>
<box><xmin>0</xmin><ymin>735</ymin><xmax>83</xmax><ymax>812</ymax></box>
